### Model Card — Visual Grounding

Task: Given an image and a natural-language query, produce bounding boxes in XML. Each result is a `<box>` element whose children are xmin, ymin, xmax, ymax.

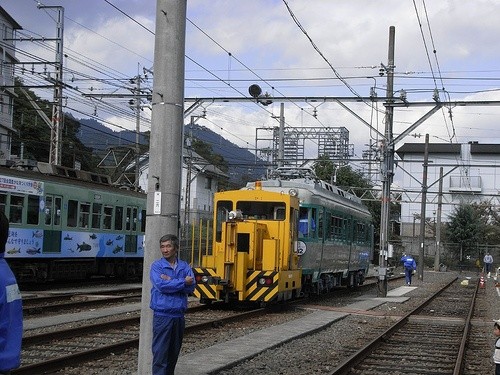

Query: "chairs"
<box><xmin>298</xmin><ymin>217</ymin><xmax>315</xmax><ymax>236</ymax></box>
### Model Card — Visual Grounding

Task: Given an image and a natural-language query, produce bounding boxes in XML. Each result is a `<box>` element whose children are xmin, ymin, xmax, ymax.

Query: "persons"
<box><xmin>0</xmin><ymin>211</ymin><xmax>23</xmax><ymax>375</ymax></box>
<box><xmin>493</xmin><ymin>321</ymin><xmax>500</xmax><ymax>375</ymax></box>
<box><xmin>298</xmin><ymin>210</ymin><xmax>316</xmax><ymax>237</ymax></box>
<box><xmin>401</xmin><ymin>252</ymin><xmax>417</xmax><ymax>286</ymax></box>
<box><xmin>475</xmin><ymin>252</ymin><xmax>493</xmax><ymax>277</ymax></box>
<box><xmin>149</xmin><ymin>233</ymin><xmax>196</xmax><ymax>375</ymax></box>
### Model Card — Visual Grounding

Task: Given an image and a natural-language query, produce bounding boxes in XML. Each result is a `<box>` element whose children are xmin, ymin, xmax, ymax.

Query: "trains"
<box><xmin>0</xmin><ymin>165</ymin><xmax>148</xmax><ymax>289</ymax></box>
<box><xmin>192</xmin><ymin>167</ymin><xmax>374</xmax><ymax>309</ymax></box>
